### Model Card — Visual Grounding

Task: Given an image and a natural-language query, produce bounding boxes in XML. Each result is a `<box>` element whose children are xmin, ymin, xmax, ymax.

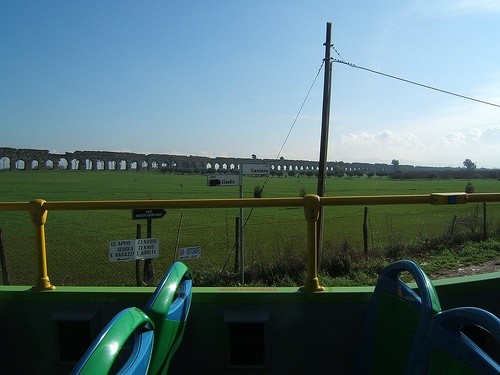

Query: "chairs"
<box><xmin>340</xmin><ymin>258</ymin><xmax>443</xmax><ymax>374</ymax></box>
<box><xmin>144</xmin><ymin>260</ymin><xmax>195</xmax><ymax>375</ymax></box>
<box><xmin>68</xmin><ymin>306</ymin><xmax>156</xmax><ymax>375</ymax></box>
<box><xmin>414</xmin><ymin>306</ymin><xmax>499</xmax><ymax>374</ymax></box>
